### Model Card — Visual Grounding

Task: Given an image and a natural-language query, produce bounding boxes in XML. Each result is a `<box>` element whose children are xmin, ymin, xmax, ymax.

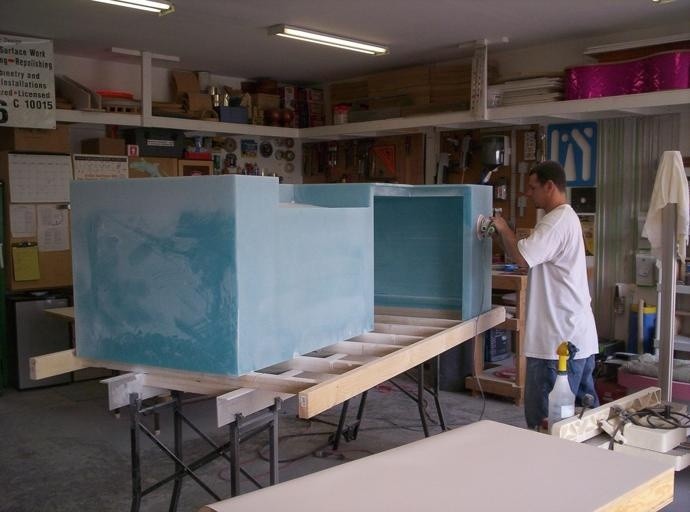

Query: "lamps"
<box><xmin>92</xmin><ymin>0</ymin><xmax>175</xmax><ymax>17</ymax></box>
<box><xmin>267</xmin><ymin>24</ymin><xmax>391</xmax><ymax>57</ymax></box>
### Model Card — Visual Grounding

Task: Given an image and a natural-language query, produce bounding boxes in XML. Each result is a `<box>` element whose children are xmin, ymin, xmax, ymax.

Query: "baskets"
<box><xmin>252</xmin><ymin>93</ymin><xmax>281</xmax><ymax>111</ymax></box>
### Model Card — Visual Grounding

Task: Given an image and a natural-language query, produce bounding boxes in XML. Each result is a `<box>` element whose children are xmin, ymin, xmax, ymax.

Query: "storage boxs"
<box><xmin>0</xmin><ymin>124</ymin><xmax>71</xmax><ymax>154</ymax></box>
<box><xmin>81</xmin><ymin>137</ymin><xmax>125</xmax><ymax>155</ymax></box>
<box><xmin>242</xmin><ymin>78</ymin><xmax>326</xmax><ymax>129</ymax></box>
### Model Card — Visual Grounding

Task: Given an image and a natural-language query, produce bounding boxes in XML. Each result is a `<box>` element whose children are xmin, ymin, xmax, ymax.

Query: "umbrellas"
<box><xmin>487</xmin><ymin>158</ymin><xmax>600</xmax><ymax>435</ymax></box>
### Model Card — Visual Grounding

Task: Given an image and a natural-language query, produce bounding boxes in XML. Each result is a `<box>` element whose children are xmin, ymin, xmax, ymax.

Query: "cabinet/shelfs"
<box><xmin>5</xmin><ymin>286</ymin><xmax>113</xmax><ymax>391</ymax></box>
<box><xmin>99</xmin><ymin>354</ymin><xmax>446</xmax><ymax>512</ymax></box>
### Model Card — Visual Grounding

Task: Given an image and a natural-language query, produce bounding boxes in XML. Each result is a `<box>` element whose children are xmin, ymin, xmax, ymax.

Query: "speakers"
<box><xmin>571</xmin><ymin>187</ymin><xmax>596</xmax><ymax>213</ymax></box>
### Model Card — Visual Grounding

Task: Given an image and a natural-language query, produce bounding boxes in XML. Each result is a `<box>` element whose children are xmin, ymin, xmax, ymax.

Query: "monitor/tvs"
<box><xmin>179</xmin><ymin>159</ymin><xmax>214</xmax><ymax>176</ymax></box>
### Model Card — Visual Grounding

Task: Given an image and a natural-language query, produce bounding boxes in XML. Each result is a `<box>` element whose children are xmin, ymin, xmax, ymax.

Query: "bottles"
<box><xmin>547</xmin><ymin>370</ymin><xmax>576</xmax><ymax>434</ymax></box>
<box><xmin>332</xmin><ymin>103</ymin><xmax>349</xmax><ymax>126</ymax></box>
